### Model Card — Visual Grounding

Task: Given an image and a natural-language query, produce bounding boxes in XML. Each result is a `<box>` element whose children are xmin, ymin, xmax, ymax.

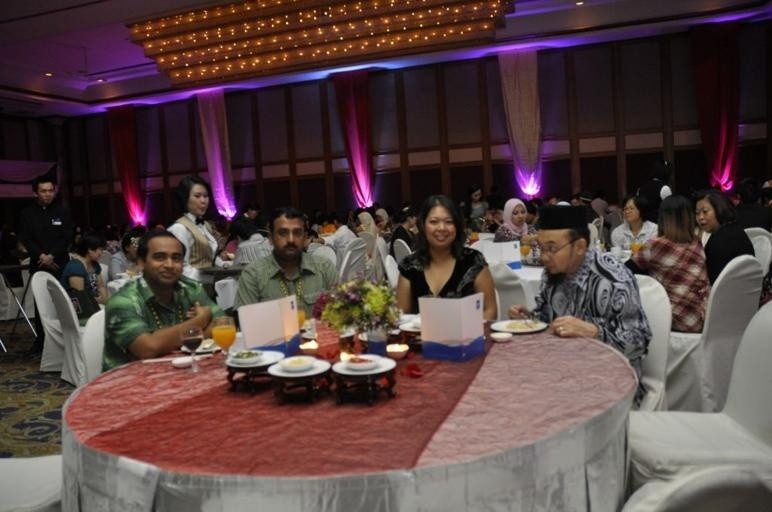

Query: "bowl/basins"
<box><xmin>229</xmin><ymin>349</ymin><xmax>265</xmax><ymax>363</ymax></box>
<box><xmin>345</xmin><ymin>354</ymin><xmax>382</xmax><ymax>370</ymax></box>
<box><xmin>278</xmin><ymin>353</ymin><xmax>314</xmax><ymax>373</ymax></box>
<box><xmin>385</xmin><ymin>343</ymin><xmax>411</xmax><ymax>359</ymax></box>
<box><xmin>298</xmin><ymin>342</ymin><xmax>320</xmax><ymax>353</ymax></box>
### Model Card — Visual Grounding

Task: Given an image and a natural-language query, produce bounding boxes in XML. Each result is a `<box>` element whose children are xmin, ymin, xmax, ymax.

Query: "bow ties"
<box><xmin>194</xmin><ymin>217</ymin><xmax>206</xmax><ymax>226</ymax></box>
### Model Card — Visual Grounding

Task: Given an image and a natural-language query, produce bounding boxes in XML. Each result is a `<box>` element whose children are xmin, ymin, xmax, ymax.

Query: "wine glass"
<box><xmin>179</xmin><ymin>322</ymin><xmax>204</xmax><ymax>373</ymax></box>
<box><xmin>210</xmin><ymin>315</ymin><xmax>236</xmax><ymax>349</ymax></box>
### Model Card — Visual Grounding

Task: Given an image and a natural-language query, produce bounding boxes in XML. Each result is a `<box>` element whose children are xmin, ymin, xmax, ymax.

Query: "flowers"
<box><xmin>312</xmin><ymin>264</ymin><xmax>400</xmax><ymax>334</ymax></box>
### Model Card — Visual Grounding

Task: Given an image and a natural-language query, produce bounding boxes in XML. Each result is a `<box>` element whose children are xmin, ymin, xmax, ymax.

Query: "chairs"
<box><xmin>629</xmin><ymin>301</ymin><xmax>772</xmax><ymax>490</ymax></box>
<box><xmin>0</xmin><ymin>453</ymin><xmax>62</xmax><ymax>511</ymax></box>
<box><xmin>630</xmin><ymin>274</ymin><xmax>674</xmax><ymax>410</ymax></box>
<box><xmin>2</xmin><ymin>216</ymin><xmax>771</xmax><ymax>321</ymax></box>
<box><xmin>665</xmin><ymin>254</ymin><xmax>765</xmax><ymax>413</ymax></box>
<box><xmin>80</xmin><ymin>307</ymin><xmax>107</xmax><ymax>387</ymax></box>
<box><xmin>46</xmin><ymin>277</ymin><xmax>85</xmax><ymax>388</ymax></box>
<box><xmin>625</xmin><ymin>468</ymin><xmax>771</xmax><ymax>510</ymax></box>
<box><xmin>30</xmin><ymin>270</ymin><xmax>85</xmax><ymax>372</ymax></box>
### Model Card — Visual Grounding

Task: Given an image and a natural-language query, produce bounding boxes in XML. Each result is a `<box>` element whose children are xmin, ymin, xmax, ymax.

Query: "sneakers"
<box><xmin>30</xmin><ymin>338</ymin><xmax>44</xmax><ymax>357</ymax></box>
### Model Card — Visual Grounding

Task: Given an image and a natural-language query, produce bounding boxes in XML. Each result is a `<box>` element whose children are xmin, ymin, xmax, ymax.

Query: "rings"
<box><xmin>560</xmin><ymin>327</ymin><xmax>563</xmax><ymax>331</ymax></box>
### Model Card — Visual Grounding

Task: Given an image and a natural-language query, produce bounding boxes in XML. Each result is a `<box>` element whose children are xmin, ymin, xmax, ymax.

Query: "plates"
<box><xmin>490</xmin><ymin>331</ymin><xmax>512</xmax><ymax>344</ymax></box>
<box><xmin>182</xmin><ymin>336</ymin><xmax>220</xmax><ymax>355</ymax></box>
<box><xmin>397</xmin><ymin>320</ymin><xmax>421</xmax><ymax>332</ymax></box>
<box><xmin>269</xmin><ymin>358</ymin><xmax>332</xmax><ymax>377</ymax></box>
<box><xmin>488</xmin><ymin>318</ymin><xmax>549</xmax><ymax>335</ymax></box>
<box><xmin>331</xmin><ymin>355</ymin><xmax>398</xmax><ymax>375</ymax></box>
<box><xmin>297</xmin><ymin>326</ymin><xmax>357</xmax><ymax>342</ymax></box>
<box><xmin>224</xmin><ymin>348</ymin><xmax>284</xmax><ymax>368</ymax></box>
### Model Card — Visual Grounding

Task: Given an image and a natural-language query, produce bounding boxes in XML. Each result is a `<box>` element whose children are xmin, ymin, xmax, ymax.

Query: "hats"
<box><xmin>537</xmin><ymin>205</ymin><xmax>589</xmax><ymax>230</ymax></box>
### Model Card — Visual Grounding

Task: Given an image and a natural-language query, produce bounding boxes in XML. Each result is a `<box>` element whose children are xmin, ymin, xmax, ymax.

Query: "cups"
<box><xmin>593</xmin><ymin>235</ymin><xmax>642</xmax><ymax>260</ymax></box>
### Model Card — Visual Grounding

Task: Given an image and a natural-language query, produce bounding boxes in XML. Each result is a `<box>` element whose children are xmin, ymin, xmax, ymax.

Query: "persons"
<box><xmin>495</xmin><ymin>194</ymin><xmax>623</xmax><ymax>251</ymax></box>
<box><xmin>355</xmin><ymin>184</ymin><xmax>498</xmax><ymax>320</ymax></box>
<box><xmin>506</xmin><ymin>205</ymin><xmax>650</xmax><ymax>376</ymax></box>
<box><xmin>610</xmin><ymin>188</ymin><xmax>755</xmax><ymax>333</ymax></box>
<box><xmin>18</xmin><ymin>175</ymin><xmax>358</xmax><ymax>371</ymax></box>
